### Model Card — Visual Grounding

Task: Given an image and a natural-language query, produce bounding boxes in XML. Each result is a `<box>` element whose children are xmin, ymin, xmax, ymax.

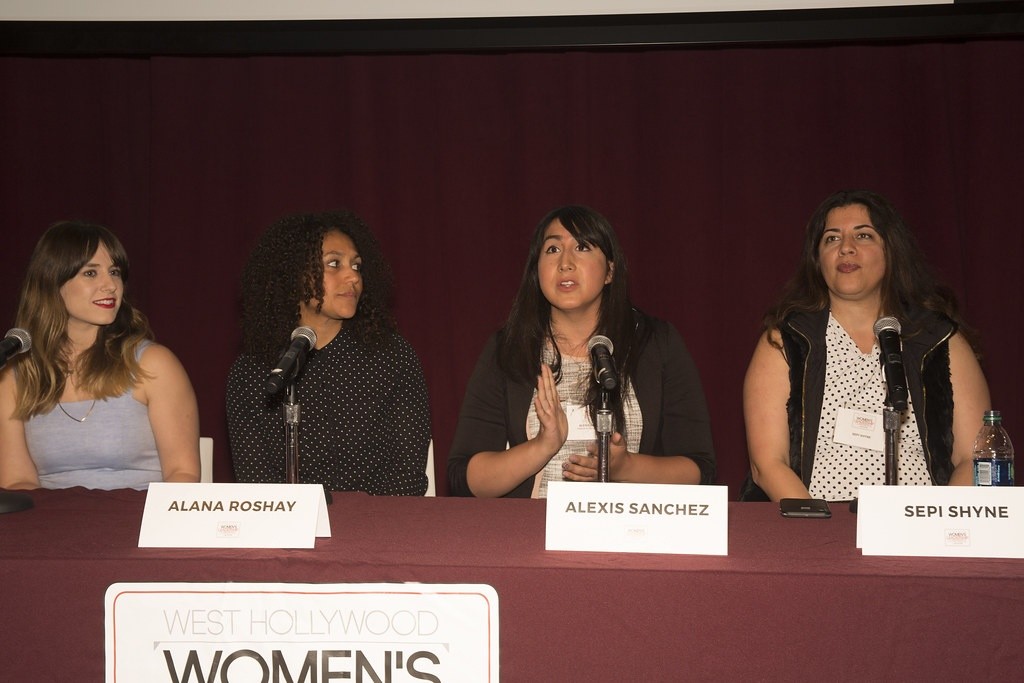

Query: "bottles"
<box><xmin>973</xmin><ymin>408</ymin><xmax>1015</xmax><ymax>488</ymax></box>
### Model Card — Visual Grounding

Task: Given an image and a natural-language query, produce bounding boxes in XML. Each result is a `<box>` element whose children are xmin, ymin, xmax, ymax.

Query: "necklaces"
<box><xmin>57</xmin><ymin>396</ymin><xmax>98</xmax><ymax>423</ymax></box>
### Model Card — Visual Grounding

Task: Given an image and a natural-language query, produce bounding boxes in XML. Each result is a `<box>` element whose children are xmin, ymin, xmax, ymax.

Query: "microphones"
<box><xmin>588</xmin><ymin>336</ymin><xmax>618</xmax><ymax>389</ymax></box>
<box><xmin>0</xmin><ymin>328</ymin><xmax>32</xmax><ymax>368</ymax></box>
<box><xmin>266</xmin><ymin>325</ymin><xmax>318</xmax><ymax>394</ymax></box>
<box><xmin>872</xmin><ymin>316</ymin><xmax>908</xmax><ymax>411</ymax></box>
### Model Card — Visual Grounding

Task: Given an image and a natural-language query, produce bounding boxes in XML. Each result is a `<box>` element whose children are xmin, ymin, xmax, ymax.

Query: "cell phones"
<box><xmin>780</xmin><ymin>498</ymin><xmax>832</xmax><ymax>518</ymax></box>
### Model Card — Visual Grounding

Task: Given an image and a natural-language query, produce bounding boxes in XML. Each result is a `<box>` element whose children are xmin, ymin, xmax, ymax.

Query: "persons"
<box><xmin>743</xmin><ymin>189</ymin><xmax>994</xmax><ymax>504</ymax></box>
<box><xmin>223</xmin><ymin>212</ymin><xmax>432</xmax><ymax>497</ymax></box>
<box><xmin>1</xmin><ymin>219</ymin><xmax>202</xmax><ymax>490</ymax></box>
<box><xmin>446</xmin><ymin>203</ymin><xmax>719</xmax><ymax>499</ymax></box>
